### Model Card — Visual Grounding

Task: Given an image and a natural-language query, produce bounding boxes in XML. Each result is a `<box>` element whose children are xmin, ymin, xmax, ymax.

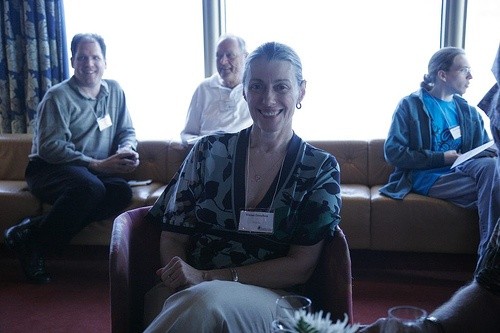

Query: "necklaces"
<box><xmin>248</xmin><ymin>142</ymin><xmax>285</xmax><ymax>183</ymax></box>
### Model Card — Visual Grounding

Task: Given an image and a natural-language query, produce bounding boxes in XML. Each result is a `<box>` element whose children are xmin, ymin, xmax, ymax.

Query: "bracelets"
<box><xmin>229</xmin><ymin>266</ymin><xmax>239</xmax><ymax>282</ymax></box>
<box><xmin>426</xmin><ymin>316</ymin><xmax>445</xmax><ymax>333</ymax></box>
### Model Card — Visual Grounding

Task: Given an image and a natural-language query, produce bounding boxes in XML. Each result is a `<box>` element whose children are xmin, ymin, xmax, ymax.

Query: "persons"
<box><xmin>4</xmin><ymin>32</ymin><xmax>140</xmax><ymax>282</ymax></box>
<box><xmin>142</xmin><ymin>42</ymin><xmax>342</xmax><ymax>333</ymax></box>
<box><xmin>357</xmin><ymin>47</ymin><xmax>500</xmax><ymax>333</ymax></box>
<box><xmin>181</xmin><ymin>35</ymin><xmax>255</xmax><ymax>150</ymax></box>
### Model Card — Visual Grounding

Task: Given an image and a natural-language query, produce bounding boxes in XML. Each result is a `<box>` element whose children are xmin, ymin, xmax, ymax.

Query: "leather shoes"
<box><xmin>4</xmin><ymin>217</ymin><xmax>32</xmax><ymax>246</ymax></box>
<box><xmin>27</xmin><ymin>251</ymin><xmax>52</xmax><ymax>282</ymax></box>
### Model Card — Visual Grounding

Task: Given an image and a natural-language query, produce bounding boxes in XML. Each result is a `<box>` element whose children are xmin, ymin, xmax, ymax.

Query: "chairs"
<box><xmin>108</xmin><ymin>204</ymin><xmax>352</xmax><ymax>333</ymax></box>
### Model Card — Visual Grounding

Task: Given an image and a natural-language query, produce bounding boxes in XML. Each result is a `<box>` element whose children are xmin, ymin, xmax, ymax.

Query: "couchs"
<box><xmin>0</xmin><ymin>132</ymin><xmax>481</xmax><ymax>288</ymax></box>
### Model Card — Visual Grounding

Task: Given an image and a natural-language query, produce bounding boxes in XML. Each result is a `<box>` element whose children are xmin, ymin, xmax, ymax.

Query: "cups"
<box><xmin>382</xmin><ymin>306</ymin><xmax>427</xmax><ymax>333</ymax></box>
<box><xmin>274</xmin><ymin>295</ymin><xmax>311</xmax><ymax>330</ymax></box>
